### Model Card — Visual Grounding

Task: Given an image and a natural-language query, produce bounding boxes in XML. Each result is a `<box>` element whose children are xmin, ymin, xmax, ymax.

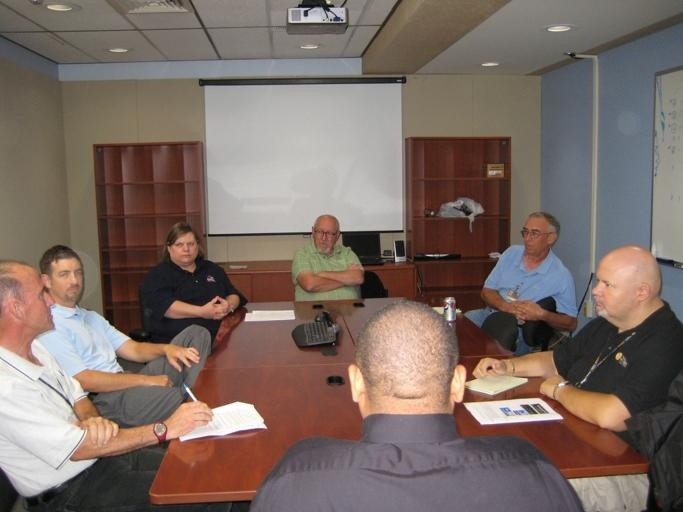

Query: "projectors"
<box><xmin>286</xmin><ymin>8</ymin><xmax>348</xmax><ymax>35</ymax></box>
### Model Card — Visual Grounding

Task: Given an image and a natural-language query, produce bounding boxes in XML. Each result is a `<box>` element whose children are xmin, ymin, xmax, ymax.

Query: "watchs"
<box><xmin>152</xmin><ymin>419</ymin><xmax>167</xmax><ymax>444</ymax></box>
<box><xmin>552</xmin><ymin>379</ymin><xmax>570</xmax><ymax>401</ymax></box>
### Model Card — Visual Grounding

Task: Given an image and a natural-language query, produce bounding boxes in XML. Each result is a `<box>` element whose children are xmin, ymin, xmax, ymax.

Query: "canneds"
<box><xmin>443</xmin><ymin>296</ymin><xmax>457</xmax><ymax>322</ymax></box>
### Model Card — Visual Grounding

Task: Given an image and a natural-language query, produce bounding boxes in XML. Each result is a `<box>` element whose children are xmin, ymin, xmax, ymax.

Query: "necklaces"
<box><xmin>575</xmin><ymin>329</ymin><xmax>637</xmax><ymax>389</ymax></box>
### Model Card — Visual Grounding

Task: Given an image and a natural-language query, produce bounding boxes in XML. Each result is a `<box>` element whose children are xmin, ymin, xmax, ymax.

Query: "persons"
<box><xmin>35</xmin><ymin>243</ymin><xmax>213</xmax><ymax>429</ymax></box>
<box><xmin>462</xmin><ymin>210</ymin><xmax>579</xmax><ymax>358</ymax></box>
<box><xmin>0</xmin><ymin>257</ymin><xmax>233</xmax><ymax>511</ymax></box>
<box><xmin>245</xmin><ymin>297</ymin><xmax>587</xmax><ymax>512</ymax></box>
<box><xmin>289</xmin><ymin>214</ymin><xmax>365</xmax><ymax>302</ymax></box>
<box><xmin>138</xmin><ymin>221</ymin><xmax>248</xmax><ymax>345</ymax></box>
<box><xmin>470</xmin><ymin>244</ymin><xmax>682</xmax><ymax>455</ymax></box>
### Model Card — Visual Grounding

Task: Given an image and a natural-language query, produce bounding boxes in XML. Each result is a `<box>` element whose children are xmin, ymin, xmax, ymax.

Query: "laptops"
<box><xmin>342</xmin><ymin>233</ymin><xmax>386</xmax><ymax>266</ymax></box>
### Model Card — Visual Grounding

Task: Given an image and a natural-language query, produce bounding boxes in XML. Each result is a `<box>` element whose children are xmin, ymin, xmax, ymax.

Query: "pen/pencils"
<box><xmin>181</xmin><ymin>381</ymin><xmax>201</xmax><ymax>403</ymax></box>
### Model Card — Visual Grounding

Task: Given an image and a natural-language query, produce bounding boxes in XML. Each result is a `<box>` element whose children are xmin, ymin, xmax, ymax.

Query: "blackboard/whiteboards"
<box><xmin>650</xmin><ymin>67</ymin><xmax>683</xmax><ymax>269</ymax></box>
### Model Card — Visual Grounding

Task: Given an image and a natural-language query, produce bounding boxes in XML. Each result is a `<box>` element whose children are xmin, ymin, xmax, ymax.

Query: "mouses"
<box><xmin>315</xmin><ymin>311</ymin><xmax>331</xmax><ymax>322</ymax></box>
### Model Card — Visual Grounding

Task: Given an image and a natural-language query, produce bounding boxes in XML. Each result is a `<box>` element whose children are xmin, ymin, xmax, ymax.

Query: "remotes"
<box><xmin>229</xmin><ymin>265</ymin><xmax>247</xmax><ymax>270</ymax></box>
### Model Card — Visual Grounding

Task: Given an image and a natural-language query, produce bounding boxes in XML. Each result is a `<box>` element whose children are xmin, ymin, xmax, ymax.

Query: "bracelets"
<box><xmin>504</xmin><ymin>359</ymin><xmax>515</xmax><ymax>376</ymax></box>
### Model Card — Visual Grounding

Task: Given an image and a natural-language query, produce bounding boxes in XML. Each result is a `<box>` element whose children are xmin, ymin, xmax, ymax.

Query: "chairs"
<box><xmin>358</xmin><ymin>269</ymin><xmax>388</xmax><ymax>299</ymax></box>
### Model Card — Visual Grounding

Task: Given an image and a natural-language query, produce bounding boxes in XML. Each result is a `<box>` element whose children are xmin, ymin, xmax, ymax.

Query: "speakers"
<box><xmin>394</xmin><ymin>241</ymin><xmax>406</xmax><ymax>263</ymax></box>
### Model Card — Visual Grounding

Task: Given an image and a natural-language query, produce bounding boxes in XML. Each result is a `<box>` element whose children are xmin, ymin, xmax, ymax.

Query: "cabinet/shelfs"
<box><xmin>406</xmin><ymin>136</ymin><xmax>512</xmax><ymax>313</ymax></box>
<box><xmin>92</xmin><ymin>141</ymin><xmax>207</xmax><ymax>338</ymax></box>
<box><xmin>212</xmin><ymin>257</ymin><xmax>418</xmax><ymax>306</ymax></box>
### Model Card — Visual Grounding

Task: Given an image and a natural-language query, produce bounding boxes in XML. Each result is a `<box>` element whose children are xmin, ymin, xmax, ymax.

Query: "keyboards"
<box><xmin>291</xmin><ymin>322</ymin><xmax>336</xmax><ymax>348</ymax></box>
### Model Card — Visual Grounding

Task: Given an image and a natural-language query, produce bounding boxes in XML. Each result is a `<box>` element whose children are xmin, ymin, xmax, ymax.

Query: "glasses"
<box><xmin>521</xmin><ymin>229</ymin><xmax>559</xmax><ymax>240</ymax></box>
<box><xmin>314</xmin><ymin>228</ymin><xmax>339</xmax><ymax>237</ymax></box>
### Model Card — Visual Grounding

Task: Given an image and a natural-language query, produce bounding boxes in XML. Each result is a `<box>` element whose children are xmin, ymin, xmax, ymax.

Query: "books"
<box><xmin>461</xmin><ymin>396</ymin><xmax>564</xmax><ymax>427</ymax></box>
<box><xmin>431</xmin><ymin>306</ymin><xmax>461</xmax><ymax>316</ymax></box>
<box><xmin>464</xmin><ymin>373</ymin><xmax>528</xmax><ymax>396</ymax></box>
